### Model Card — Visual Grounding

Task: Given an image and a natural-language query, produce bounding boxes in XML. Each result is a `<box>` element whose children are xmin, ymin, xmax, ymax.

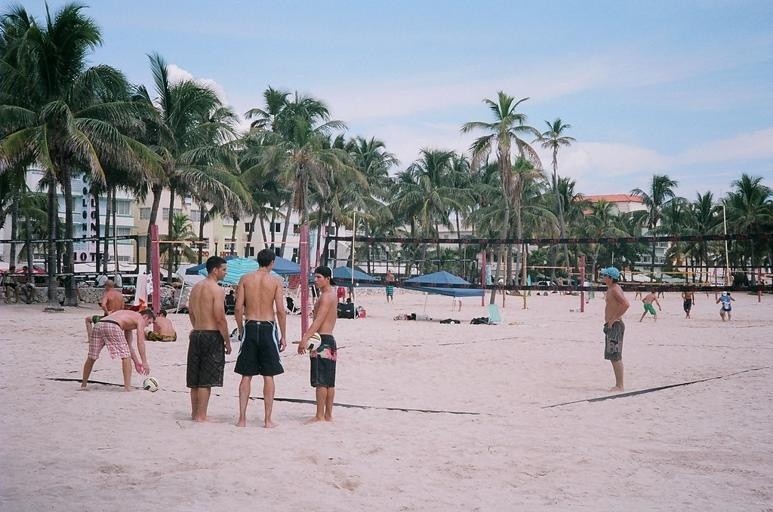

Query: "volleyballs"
<box><xmin>143</xmin><ymin>377</ymin><xmax>159</xmax><ymax>392</ymax></box>
<box><xmin>307</xmin><ymin>332</ymin><xmax>322</xmax><ymax>351</ymax></box>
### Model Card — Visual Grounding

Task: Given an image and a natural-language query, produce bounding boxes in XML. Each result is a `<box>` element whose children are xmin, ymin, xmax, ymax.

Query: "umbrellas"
<box><xmin>307</xmin><ymin>262</ymin><xmax>379</xmax><ymax>302</ymax></box>
<box><xmin>403</xmin><ymin>269</ymin><xmax>472</xmax><ymax>313</ymax></box>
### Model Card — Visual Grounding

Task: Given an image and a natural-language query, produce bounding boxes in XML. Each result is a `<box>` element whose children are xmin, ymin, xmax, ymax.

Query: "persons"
<box><xmin>298</xmin><ymin>266</ymin><xmax>338</xmax><ymax>425</ymax></box>
<box><xmin>633</xmin><ymin>282</ymin><xmax>737</xmax><ymax>322</ymax></box>
<box><xmin>144</xmin><ymin>310</ymin><xmax>177</xmax><ymax>342</ymax></box>
<box><xmin>226</xmin><ymin>290</ymin><xmax>234</xmax><ymax>309</ymax></box>
<box><xmin>600</xmin><ymin>266</ymin><xmax>631</xmax><ymax>393</ymax></box>
<box><xmin>234</xmin><ymin>249</ymin><xmax>287</xmax><ymax>428</ymax></box>
<box><xmin>95</xmin><ymin>271</ymin><xmax>122</xmax><ymax>288</ymax></box>
<box><xmin>8</xmin><ymin>264</ymin><xmax>39</xmax><ymax>276</ymax></box>
<box><xmin>83</xmin><ymin>280</ymin><xmax>124</xmax><ymax>343</ymax></box>
<box><xmin>187</xmin><ymin>256</ymin><xmax>231</xmax><ymax>422</ymax></box>
<box><xmin>81</xmin><ymin>308</ymin><xmax>156</xmax><ymax>392</ymax></box>
<box><xmin>384</xmin><ymin>270</ymin><xmax>395</xmax><ymax>303</ymax></box>
<box><xmin>337</xmin><ymin>284</ymin><xmax>355</xmax><ymax>302</ymax></box>
<box><xmin>286</xmin><ymin>273</ymin><xmax>300</xmax><ymax>314</ymax></box>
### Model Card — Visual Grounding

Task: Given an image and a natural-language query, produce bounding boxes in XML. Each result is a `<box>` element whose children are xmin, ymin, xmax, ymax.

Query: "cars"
<box><xmin>537</xmin><ymin>279</ymin><xmax>575</xmax><ymax>286</ymax></box>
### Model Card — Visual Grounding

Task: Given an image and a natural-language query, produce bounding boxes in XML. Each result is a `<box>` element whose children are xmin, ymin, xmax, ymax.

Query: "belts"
<box><xmin>98</xmin><ymin>318</ymin><xmax>121</xmax><ymax>328</ymax></box>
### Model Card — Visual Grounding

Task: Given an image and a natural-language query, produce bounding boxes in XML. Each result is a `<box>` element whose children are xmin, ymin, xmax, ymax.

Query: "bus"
<box><xmin>73</xmin><ymin>274</ymin><xmax>138</xmax><ymax>291</ymax></box>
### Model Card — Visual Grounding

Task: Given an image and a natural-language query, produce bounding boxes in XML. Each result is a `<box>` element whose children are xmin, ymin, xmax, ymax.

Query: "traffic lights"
<box><xmin>708</xmin><ymin>205</ymin><xmax>730</xmax><ymax>286</ymax></box>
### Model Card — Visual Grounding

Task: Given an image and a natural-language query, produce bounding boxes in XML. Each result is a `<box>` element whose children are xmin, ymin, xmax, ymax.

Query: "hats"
<box><xmin>315</xmin><ymin>265</ymin><xmax>336</xmax><ymax>285</ymax></box>
<box><xmin>600</xmin><ymin>266</ymin><xmax>619</xmax><ymax>279</ymax></box>
<box><xmin>722</xmin><ymin>291</ymin><xmax>727</xmax><ymax>296</ymax></box>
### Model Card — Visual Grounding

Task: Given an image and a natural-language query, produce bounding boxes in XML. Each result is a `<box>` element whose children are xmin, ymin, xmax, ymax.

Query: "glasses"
<box><xmin>156</xmin><ymin>312</ymin><xmax>161</xmax><ymax>315</ymax></box>
